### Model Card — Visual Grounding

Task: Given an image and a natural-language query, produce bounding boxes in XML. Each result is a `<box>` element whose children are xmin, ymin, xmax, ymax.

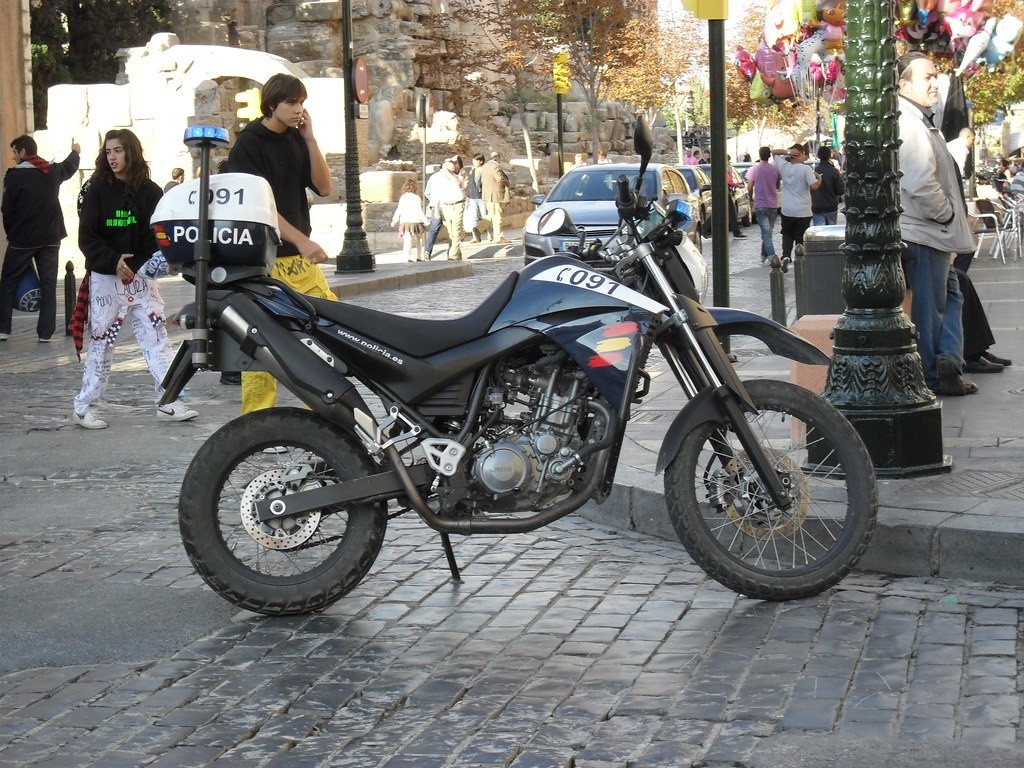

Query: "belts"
<box><xmin>444</xmin><ymin>200</ymin><xmax>464</xmax><ymax>205</ymax></box>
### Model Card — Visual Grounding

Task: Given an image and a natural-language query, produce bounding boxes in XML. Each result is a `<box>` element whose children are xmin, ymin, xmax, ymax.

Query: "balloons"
<box><xmin>734</xmin><ymin>0</ymin><xmax>1024</xmax><ymax>110</ymax></box>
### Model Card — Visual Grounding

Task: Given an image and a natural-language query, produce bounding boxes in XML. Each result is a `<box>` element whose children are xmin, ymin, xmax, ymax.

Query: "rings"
<box><xmin>123</xmin><ymin>265</ymin><xmax>127</xmax><ymax>269</ymax></box>
<box><xmin>122</xmin><ymin>269</ymin><xmax>126</xmax><ymax>273</ymax></box>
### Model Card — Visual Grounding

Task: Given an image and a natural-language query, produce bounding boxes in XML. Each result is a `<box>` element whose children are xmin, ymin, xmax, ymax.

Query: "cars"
<box><xmin>728</xmin><ymin>162</ymin><xmax>761</xmax><ymax>228</ymax></box>
<box><xmin>673</xmin><ymin>165</ymin><xmax>714</xmax><ymax>238</ymax></box>
<box><xmin>524</xmin><ymin>161</ymin><xmax>702</xmax><ymax>273</ymax></box>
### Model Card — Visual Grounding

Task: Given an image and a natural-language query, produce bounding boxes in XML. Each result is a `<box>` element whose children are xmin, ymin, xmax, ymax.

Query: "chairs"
<box><xmin>582</xmin><ymin>181</ymin><xmax>609</xmax><ymax>199</ymax></box>
<box><xmin>966</xmin><ymin>192</ymin><xmax>1024</xmax><ymax>264</ymax></box>
<box><xmin>742</xmin><ymin>170</ymin><xmax>749</xmax><ymax>181</ymax></box>
<box><xmin>631</xmin><ymin>176</ymin><xmax>655</xmax><ymax>199</ymax></box>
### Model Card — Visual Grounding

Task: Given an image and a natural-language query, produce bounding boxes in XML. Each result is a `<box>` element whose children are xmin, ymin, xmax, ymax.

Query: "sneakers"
<box><xmin>157</xmin><ymin>398</ymin><xmax>199</xmax><ymax>422</ymax></box>
<box><xmin>73</xmin><ymin>406</ymin><xmax>107</xmax><ymax>429</ymax></box>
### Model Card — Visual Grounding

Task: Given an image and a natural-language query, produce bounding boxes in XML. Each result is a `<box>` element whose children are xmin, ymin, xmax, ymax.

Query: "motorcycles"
<box><xmin>151</xmin><ymin>122</ymin><xmax>880</xmax><ymax>618</ymax></box>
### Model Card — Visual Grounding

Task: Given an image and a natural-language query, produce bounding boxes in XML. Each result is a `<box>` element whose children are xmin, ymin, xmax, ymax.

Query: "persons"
<box><xmin>745</xmin><ymin>144</ymin><xmax>844</xmax><ymax>273</ymax></box>
<box><xmin>0</xmin><ymin>134</ymin><xmax>81</xmax><ymax>343</ymax></box>
<box><xmin>895</xmin><ymin>52</ymin><xmax>1024</xmax><ymax>395</ymax></box>
<box><xmin>164</xmin><ymin>166</ymin><xmax>212</xmax><ymax>197</ymax></box>
<box><xmin>727</xmin><ymin>155</ymin><xmax>749</xmax><ymax>239</ymax></box>
<box><xmin>227</xmin><ymin>73</ymin><xmax>340</xmax><ymax>454</ymax></box>
<box><xmin>72</xmin><ymin>129</ymin><xmax>199</xmax><ymax>429</ymax></box>
<box><xmin>391</xmin><ymin>150</ymin><xmax>513</xmax><ymax>264</ymax></box>
<box><xmin>683</xmin><ymin>148</ymin><xmax>711</xmax><ymax>165</ymax></box>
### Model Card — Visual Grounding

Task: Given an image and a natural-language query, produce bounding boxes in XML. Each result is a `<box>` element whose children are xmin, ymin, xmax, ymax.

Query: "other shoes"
<box><xmin>497</xmin><ymin>238</ymin><xmax>511</xmax><ymax>243</ymax></box>
<box><xmin>964</xmin><ymin>356</ymin><xmax>1003</xmax><ymax>373</ymax></box>
<box><xmin>936</xmin><ymin>358</ymin><xmax>962</xmax><ymax>394</ymax></box>
<box><xmin>0</xmin><ymin>333</ymin><xmax>8</xmax><ymax>340</ymax></box>
<box><xmin>988</xmin><ymin>352</ymin><xmax>1012</xmax><ymax>367</ymax></box>
<box><xmin>221</xmin><ymin>375</ymin><xmax>241</xmax><ymax>385</ymax></box>
<box><xmin>781</xmin><ymin>257</ymin><xmax>790</xmax><ymax>273</ymax></box>
<box><xmin>423</xmin><ymin>253</ymin><xmax>431</xmax><ymax>260</ymax></box>
<box><xmin>474</xmin><ymin>227</ymin><xmax>481</xmax><ymax>241</ymax></box>
<box><xmin>931</xmin><ymin>382</ymin><xmax>977</xmax><ymax>394</ymax></box>
<box><xmin>39</xmin><ymin>337</ymin><xmax>52</xmax><ymax>342</ymax></box>
<box><xmin>733</xmin><ymin>233</ymin><xmax>748</xmax><ymax>240</ymax></box>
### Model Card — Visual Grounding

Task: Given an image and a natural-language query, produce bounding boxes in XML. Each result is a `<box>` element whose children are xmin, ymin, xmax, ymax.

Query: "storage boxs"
<box><xmin>147</xmin><ymin>172</ymin><xmax>283</xmax><ymax>286</ymax></box>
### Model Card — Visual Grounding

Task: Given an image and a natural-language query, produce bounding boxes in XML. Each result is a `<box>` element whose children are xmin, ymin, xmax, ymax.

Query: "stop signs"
<box><xmin>425</xmin><ymin>94</ymin><xmax>434</xmax><ymax>126</ymax></box>
